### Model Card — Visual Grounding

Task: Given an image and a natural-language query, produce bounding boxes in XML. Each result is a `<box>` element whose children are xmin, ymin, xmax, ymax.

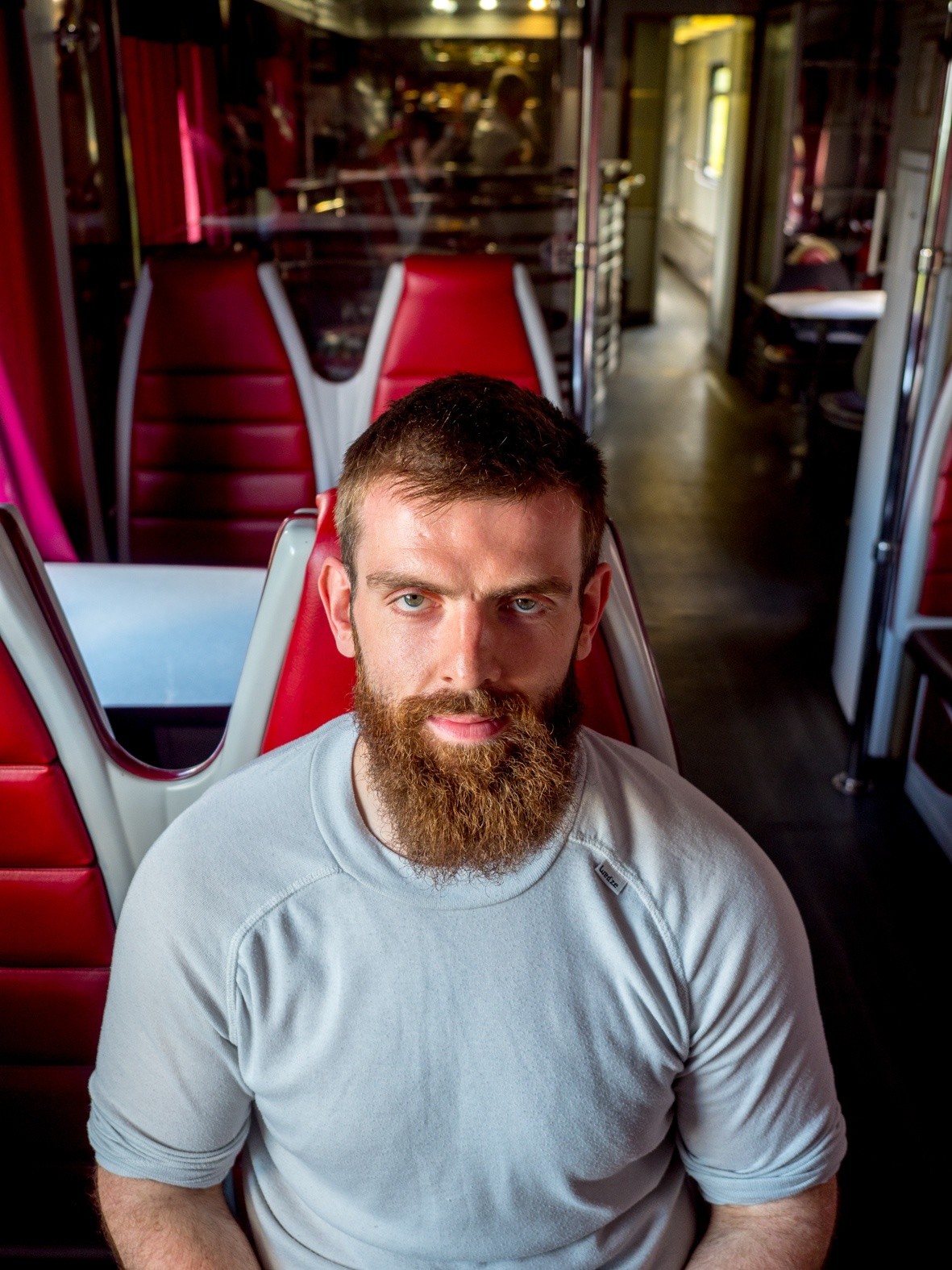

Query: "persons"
<box><xmin>399</xmin><ymin>90</ymin><xmax>457</xmax><ymax>192</ymax></box>
<box><xmin>470</xmin><ymin>74</ymin><xmax>539</xmax><ymax>170</ymax></box>
<box><xmin>86</xmin><ymin>373</ymin><xmax>849</xmax><ymax>1270</ymax></box>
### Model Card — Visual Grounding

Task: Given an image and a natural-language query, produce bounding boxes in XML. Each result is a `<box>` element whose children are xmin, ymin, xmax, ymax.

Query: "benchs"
<box><xmin>859</xmin><ymin>362</ymin><xmax>952</xmax><ymax>863</ymax></box>
<box><xmin>0</xmin><ymin>242</ymin><xmax>676</xmax><ymax>1267</ymax></box>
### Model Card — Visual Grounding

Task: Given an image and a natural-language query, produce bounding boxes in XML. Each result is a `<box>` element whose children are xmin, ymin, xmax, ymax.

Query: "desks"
<box><xmin>764</xmin><ymin>279</ymin><xmax>885</xmax><ymax>484</ymax></box>
